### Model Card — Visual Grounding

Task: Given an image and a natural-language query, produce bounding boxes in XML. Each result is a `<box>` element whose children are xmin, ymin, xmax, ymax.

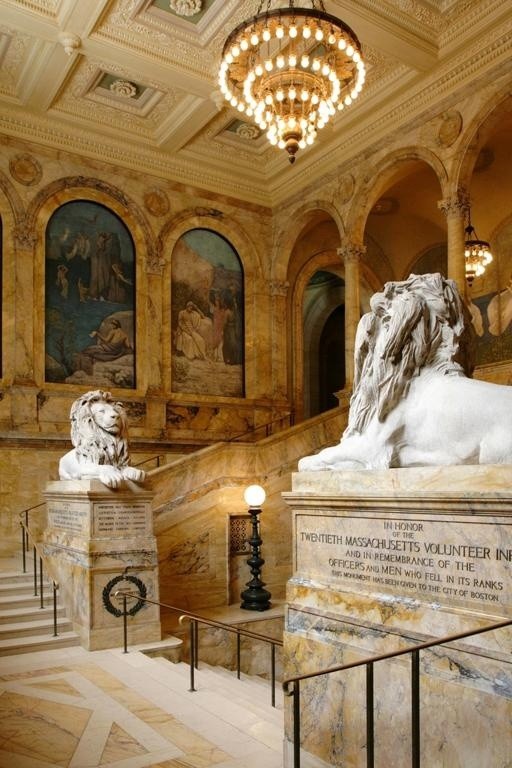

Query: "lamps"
<box><xmin>465</xmin><ymin>182</ymin><xmax>495</xmax><ymax>289</ymax></box>
<box><xmin>220</xmin><ymin>0</ymin><xmax>367</xmax><ymax>166</ymax></box>
<box><xmin>239</xmin><ymin>484</ymin><xmax>272</xmax><ymax>612</ymax></box>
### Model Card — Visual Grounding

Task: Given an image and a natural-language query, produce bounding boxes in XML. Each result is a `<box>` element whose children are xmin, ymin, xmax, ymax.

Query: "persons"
<box><xmin>64</xmin><ymin>231</ymin><xmax>91</xmax><ymax>303</ymax></box>
<box><xmin>173</xmin><ymin>262</ymin><xmax>235</xmax><ymax>364</ymax></box>
<box><xmin>91</xmin><ymin>232</ymin><xmax>121</xmax><ymax>304</ymax></box>
<box><xmin>87</xmin><ymin>319</ymin><xmax>131</xmax><ymax>365</ymax></box>
